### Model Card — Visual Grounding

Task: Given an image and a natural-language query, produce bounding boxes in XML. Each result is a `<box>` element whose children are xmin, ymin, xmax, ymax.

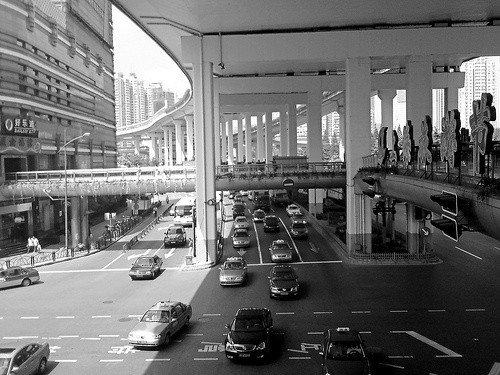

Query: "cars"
<box><xmin>289</xmin><ymin>219</ymin><xmax>309</xmax><ymax>237</ymax></box>
<box><xmin>229</xmin><ymin>190</ymin><xmax>303</xmax><ymax>233</ymax></box>
<box><xmin>268</xmin><ymin>239</ymin><xmax>294</xmax><ymax>263</ymax></box>
<box><xmin>164</xmin><ymin>225</ymin><xmax>186</xmax><ymax>247</ymax></box>
<box><xmin>318</xmin><ymin>327</ymin><xmax>371</xmax><ymax>375</ymax></box>
<box><xmin>127</xmin><ymin>301</ymin><xmax>193</xmax><ymax>348</ymax></box>
<box><xmin>267</xmin><ymin>265</ymin><xmax>300</xmax><ymax>299</ymax></box>
<box><xmin>0</xmin><ymin>266</ymin><xmax>40</xmax><ymax>289</ymax></box>
<box><xmin>230</xmin><ymin>229</ymin><xmax>251</xmax><ymax>248</ymax></box>
<box><xmin>0</xmin><ymin>342</ymin><xmax>50</xmax><ymax>375</ymax></box>
<box><xmin>129</xmin><ymin>254</ymin><xmax>163</xmax><ymax>280</ymax></box>
<box><xmin>224</xmin><ymin>308</ymin><xmax>274</xmax><ymax>363</ymax></box>
<box><xmin>219</xmin><ymin>256</ymin><xmax>248</xmax><ymax>287</ymax></box>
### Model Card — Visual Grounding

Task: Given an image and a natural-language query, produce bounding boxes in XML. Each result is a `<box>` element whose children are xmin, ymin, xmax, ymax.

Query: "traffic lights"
<box><xmin>361</xmin><ymin>177</ymin><xmax>376</xmax><ymax>186</ymax></box>
<box><xmin>362</xmin><ymin>189</ymin><xmax>375</xmax><ymax>198</ymax></box>
<box><xmin>430</xmin><ymin>190</ymin><xmax>458</xmax><ymax>217</ymax></box>
<box><xmin>430</xmin><ymin>214</ymin><xmax>463</xmax><ymax>243</ymax></box>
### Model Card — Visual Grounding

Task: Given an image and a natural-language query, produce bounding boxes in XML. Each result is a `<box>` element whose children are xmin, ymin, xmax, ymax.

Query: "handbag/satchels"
<box><xmin>37</xmin><ymin>245</ymin><xmax>41</xmax><ymax>251</ymax></box>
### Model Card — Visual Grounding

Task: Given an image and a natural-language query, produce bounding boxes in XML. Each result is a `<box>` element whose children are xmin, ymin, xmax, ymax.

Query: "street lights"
<box><xmin>57</xmin><ymin>127</ymin><xmax>91</xmax><ymax>258</ymax></box>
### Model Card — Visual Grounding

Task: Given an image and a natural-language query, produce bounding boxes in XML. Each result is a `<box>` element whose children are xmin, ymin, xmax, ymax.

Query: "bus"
<box><xmin>174</xmin><ymin>196</ymin><xmax>196</xmax><ymax>226</ymax></box>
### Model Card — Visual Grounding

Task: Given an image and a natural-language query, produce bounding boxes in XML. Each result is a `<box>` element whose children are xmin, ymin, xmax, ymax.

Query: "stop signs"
<box><xmin>282</xmin><ymin>178</ymin><xmax>295</xmax><ymax>190</ymax></box>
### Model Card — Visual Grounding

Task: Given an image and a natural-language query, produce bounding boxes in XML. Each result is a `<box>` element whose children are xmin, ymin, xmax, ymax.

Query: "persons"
<box><xmin>28</xmin><ymin>234</ymin><xmax>39</xmax><ymax>253</ymax></box>
<box><xmin>270</xmin><ymin>158</ymin><xmax>278</xmax><ymax>172</ymax></box>
<box><xmin>166</xmin><ymin>195</ymin><xmax>169</xmax><ymax>203</ymax></box>
<box><xmin>257</xmin><ymin>160</ymin><xmax>262</xmax><ymax>164</ymax></box>
<box><xmin>163</xmin><ymin>312</ymin><xmax>169</xmax><ymax>320</ymax></box>
<box><xmin>188</xmin><ymin>238</ymin><xmax>193</xmax><ymax>256</ymax></box>
<box><xmin>153</xmin><ymin>206</ymin><xmax>158</xmax><ymax>217</ymax></box>
